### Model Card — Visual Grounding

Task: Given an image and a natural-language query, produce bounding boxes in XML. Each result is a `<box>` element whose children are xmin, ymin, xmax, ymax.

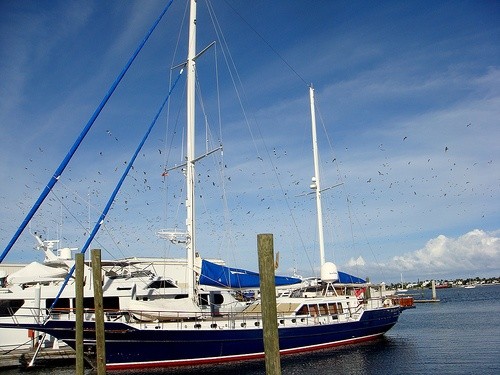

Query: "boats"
<box><xmin>0</xmin><ymin>0</ymin><xmax>416</xmax><ymax>375</ymax></box>
